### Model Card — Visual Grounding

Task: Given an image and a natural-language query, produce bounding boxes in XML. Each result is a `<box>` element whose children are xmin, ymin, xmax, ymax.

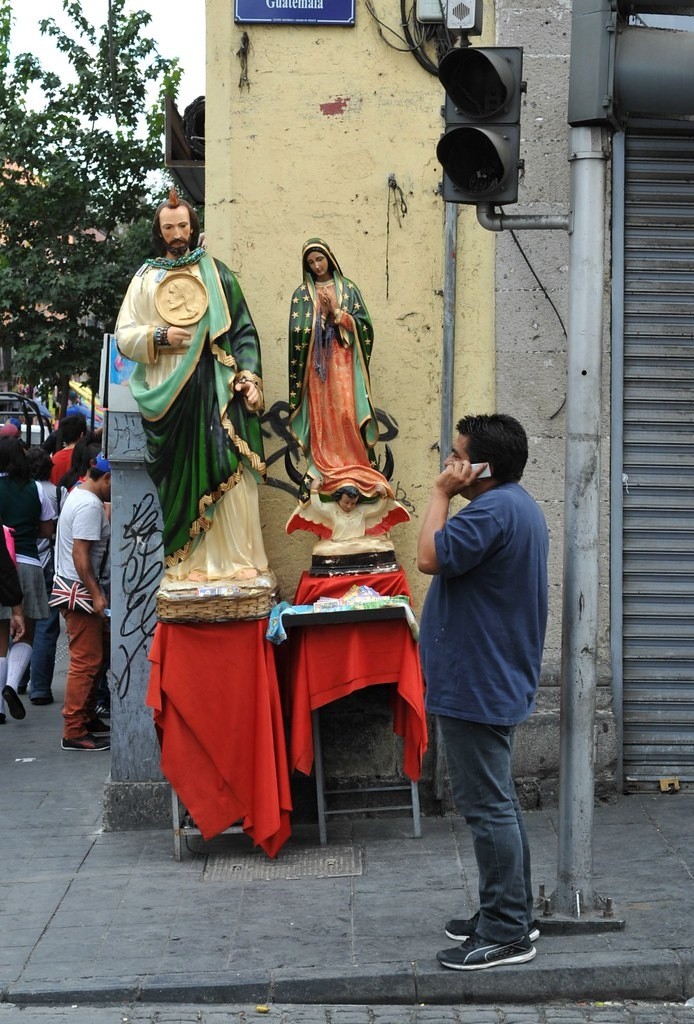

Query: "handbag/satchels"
<box><xmin>48</xmin><ymin>574</ymin><xmax>100</xmax><ymax>615</ymax></box>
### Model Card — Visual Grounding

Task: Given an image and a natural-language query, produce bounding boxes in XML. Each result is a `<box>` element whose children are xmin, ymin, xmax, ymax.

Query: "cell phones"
<box><xmin>470</xmin><ymin>463</ymin><xmax>492</xmax><ymax>479</ymax></box>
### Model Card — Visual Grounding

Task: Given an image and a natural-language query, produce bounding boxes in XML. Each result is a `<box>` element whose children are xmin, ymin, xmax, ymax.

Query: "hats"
<box><xmin>0</xmin><ymin>424</ymin><xmax>19</xmax><ymax>438</ymax></box>
<box><xmin>6</xmin><ymin>419</ymin><xmax>21</xmax><ymax>431</ymax></box>
<box><xmin>94</xmin><ymin>450</ymin><xmax>111</xmax><ymax>473</ymax></box>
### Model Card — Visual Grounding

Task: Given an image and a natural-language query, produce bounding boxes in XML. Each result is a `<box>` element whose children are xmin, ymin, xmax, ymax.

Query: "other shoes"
<box><xmin>2</xmin><ymin>685</ymin><xmax>26</xmax><ymax>720</ymax></box>
<box><xmin>32</xmin><ymin>698</ymin><xmax>54</xmax><ymax>706</ymax></box>
<box><xmin>18</xmin><ymin>686</ymin><xmax>27</xmax><ymax>694</ymax></box>
<box><xmin>0</xmin><ymin>713</ymin><xmax>6</xmax><ymax>723</ymax></box>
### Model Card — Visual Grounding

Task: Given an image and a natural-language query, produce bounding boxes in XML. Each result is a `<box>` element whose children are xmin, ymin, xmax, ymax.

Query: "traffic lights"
<box><xmin>437</xmin><ymin>44</ymin><xmax>522</xmax><ymax>207</ymax></box>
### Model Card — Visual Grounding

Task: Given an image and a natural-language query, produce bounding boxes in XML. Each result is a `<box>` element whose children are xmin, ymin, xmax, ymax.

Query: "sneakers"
<box><xmin>61</xmin><ymin>733</ymin><xmax>110</xmax><ymax>751</ymax></box>
<box><xmin>86</xmin><ymin>719</ymin><xmax>110</xmax><ymax>735</ymax></box>
<box><xmin>94</xmin><ymin>704</ymin><xmax>112</xmax><ymax>719</ymax></box>
<box><xmin>446</xmin><ymin>910</ymin><xmax>540</xmax><ymax>945</ymax></box>
<box><xmin>436</xmin><ymin>931</ymin><xmax>537</xmax><ymax>971</ymax></box>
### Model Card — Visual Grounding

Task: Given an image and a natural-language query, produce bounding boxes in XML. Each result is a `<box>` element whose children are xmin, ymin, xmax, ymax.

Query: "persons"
<box><xmin>288</xmin><ymin>237</ymin><xmax>395</xmax><ymax>543</ymax></box>
<box><xmin>0</xmin><ymin>414</ymin><xmax>112</xmax><ymax>752</ymax></box>
<box><xmin>417</xmin><ymin>413</ymin><xmax>550</xmax><ymax>972</ymax></box>
<box><xmin>113</xmin><ymin>186</ymin><xmax>267</xmax><ymax>582</ymax></box>
